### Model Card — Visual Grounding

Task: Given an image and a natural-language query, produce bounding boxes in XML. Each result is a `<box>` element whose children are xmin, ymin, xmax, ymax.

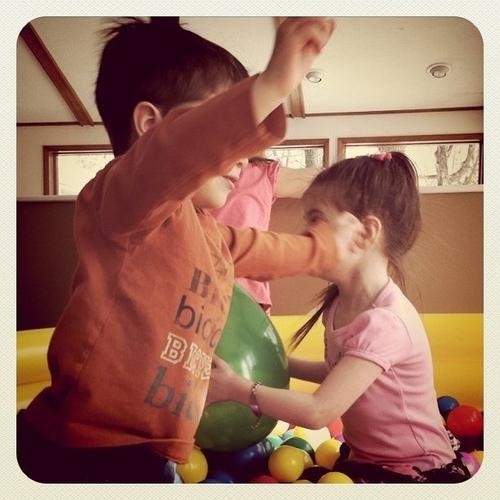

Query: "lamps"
<box><xmin>426</xmin><ymin>63</ymin><xmax>451</xmax><ymax>79</ymax></box>
<box><xmin>305</xmin><ymin>69</ymin><xmax>326</xmax><ymax>86</ymax></box>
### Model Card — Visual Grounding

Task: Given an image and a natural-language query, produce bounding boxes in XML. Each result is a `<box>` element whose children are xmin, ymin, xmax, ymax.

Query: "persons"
<box><xmin>204</xmin><ymin>149</ymin><xmax>470</xmax><ymax>483</ymax></box>
<box><xmin>197</xmin><ymin>156</ymin><xmax>284</xmax><ymax>316</ymax></box>
<box><xmin>17</xmin><ymin>17</ymin><xmax>340</xmax><ymax>487</ymax></box>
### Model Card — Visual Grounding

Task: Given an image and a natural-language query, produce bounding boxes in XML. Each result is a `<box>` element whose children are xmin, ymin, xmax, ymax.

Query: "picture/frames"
<box><xmin>43</xmin><ymin>144</ymin><xmax>115</xmax><ymax>195</ymax></box>
<box><xmin>337</xmin><ymin>132</ymin><xmax>484</xmax><ymax>186</ymax></box>
<box><xmin>264</xmin><ymin>139</ymin><xmax>330</xmax><ymax>168</ymax></box>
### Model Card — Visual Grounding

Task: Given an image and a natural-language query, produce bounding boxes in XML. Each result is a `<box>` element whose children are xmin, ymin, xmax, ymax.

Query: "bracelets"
<box><xmin>248</xmin><ymin>382</ymin><xmax>267</xmax><ymax>420</ymax></box>
